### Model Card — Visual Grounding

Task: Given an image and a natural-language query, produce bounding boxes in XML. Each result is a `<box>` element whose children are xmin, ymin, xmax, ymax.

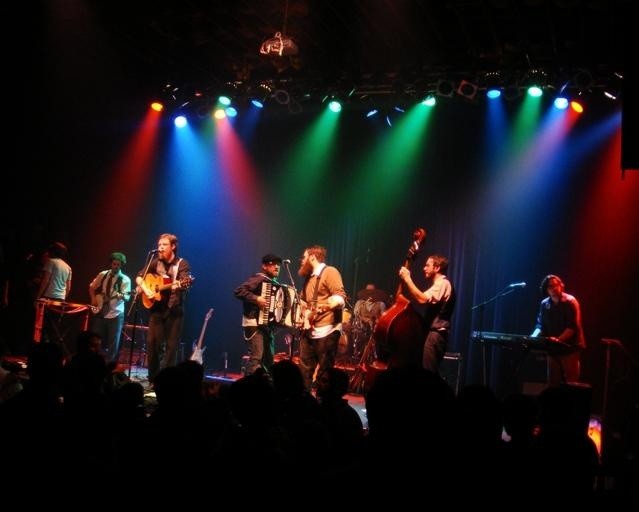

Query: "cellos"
<box><xmin>347</xmin><ymin>228</ymin><xmax>425</xmax><ymax>395</ymax></box>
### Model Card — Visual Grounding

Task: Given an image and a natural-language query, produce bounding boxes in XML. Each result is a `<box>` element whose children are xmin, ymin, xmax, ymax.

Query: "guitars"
<box><xmin>284</xmin><ymin>299</ymin><xmax>330</xmax><ymax>329</ymax></box>
<box><xmin>142</xmin><ymin>272</ymin><xmax>195</xmax><ymax>309</ymax></box>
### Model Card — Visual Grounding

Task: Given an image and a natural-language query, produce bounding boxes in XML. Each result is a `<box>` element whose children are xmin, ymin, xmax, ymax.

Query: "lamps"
<box><xmin>144</xmin><ymin>71</ymin><xmax>627</xmax><ymax>127</ymax></box>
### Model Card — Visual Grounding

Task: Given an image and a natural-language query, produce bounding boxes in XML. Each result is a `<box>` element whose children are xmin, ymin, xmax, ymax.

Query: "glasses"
<box><xmin>265</xmin><ymin>261</ymin><xmax>282</xmax><ymax>266</ymax></box>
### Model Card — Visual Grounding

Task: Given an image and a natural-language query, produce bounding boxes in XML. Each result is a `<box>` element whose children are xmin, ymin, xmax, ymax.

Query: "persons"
<box><xmin>399</xmin><ymin>256</ymin><xmax>454</xmax><ymax>378</ymax></box>
<box><xmin>353</xmin><ymin>282</ymin><xmax>387</xmax><ymax>359</ymax></box>
<box><xmin>87</xmin><ymin>251</ymin><xmax>131</xmax><ymax>361</ymax></box>
<box><xmin>528</xmin><ymin>274</ymin><xmax>587</xmax><ymax>396</ymax></box>
<box><xmin>133</xmin><ymin>233</ymin><xmax>192</xmax><ymax>395</ymax></box>
<box><xmin>232</xmin><ymin>250</ymin><xmax>284</xmax><ymax>382</ymax></box>
<box><xmin>291</xmin><ymin>246</ymin><xmax>348</xmax><ymax>409</ymax></box>
<box><xmin>33</xmin><ymin>241</ymin><xmax>73</xmax><ymax>303</ymax></box>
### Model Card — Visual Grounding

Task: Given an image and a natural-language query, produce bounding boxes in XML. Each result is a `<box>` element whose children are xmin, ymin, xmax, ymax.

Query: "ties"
<box><xmin>106</xmin><ymin>273</ymin><xmax>115</xmax><ymax>297</ymax></box>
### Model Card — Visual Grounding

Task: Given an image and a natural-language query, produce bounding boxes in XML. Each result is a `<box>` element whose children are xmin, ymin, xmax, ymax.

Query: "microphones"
<box><xmin>279</xmin><ymin>259</ymin><xmax>290</xmax><ymax>264</ymax></box>
<box><xmin>151</xmin><ymin>249</ymin><xmax>163</xmax><ymax>253</ymax></box>
<box><xmin>510</xmin><ymin>280</ymin><xmax>529</xmax><ymax>287</ymax></box>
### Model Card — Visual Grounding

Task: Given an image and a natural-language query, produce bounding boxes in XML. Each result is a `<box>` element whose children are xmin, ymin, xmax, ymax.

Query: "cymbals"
<box><xmin>356</xmin><ymin>288</ymin><xmax>387</xmax><ymax>302</ymax></box>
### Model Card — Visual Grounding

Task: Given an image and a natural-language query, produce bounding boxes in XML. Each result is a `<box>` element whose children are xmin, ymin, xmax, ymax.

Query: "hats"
<box><xmin>262</xmin><ymin>253</ymin><xmax>283</xmax><ymax>264</ymax></box>
<box><xmin>110</xmin><ymin>252</ymin><xmax>127</xmax><ymax>263</ymax></box>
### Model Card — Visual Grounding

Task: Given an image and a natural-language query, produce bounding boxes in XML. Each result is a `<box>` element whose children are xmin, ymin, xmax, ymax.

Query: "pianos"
<box><xmin>36</xmin><ymin>297</ymin><xmax>98</xmax><ymax>312</ymax></box>
<box><xmin>470</xmin><ymin>330</ymin><xmax>568</xmax><ymax>354</ymax></box>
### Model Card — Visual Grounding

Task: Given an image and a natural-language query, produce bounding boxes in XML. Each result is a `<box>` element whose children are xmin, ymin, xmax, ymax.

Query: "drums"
<box><xmin>352</xmin><ymin>313</ymin><xmax>379</xmax><ymax>338</ymax></box>
